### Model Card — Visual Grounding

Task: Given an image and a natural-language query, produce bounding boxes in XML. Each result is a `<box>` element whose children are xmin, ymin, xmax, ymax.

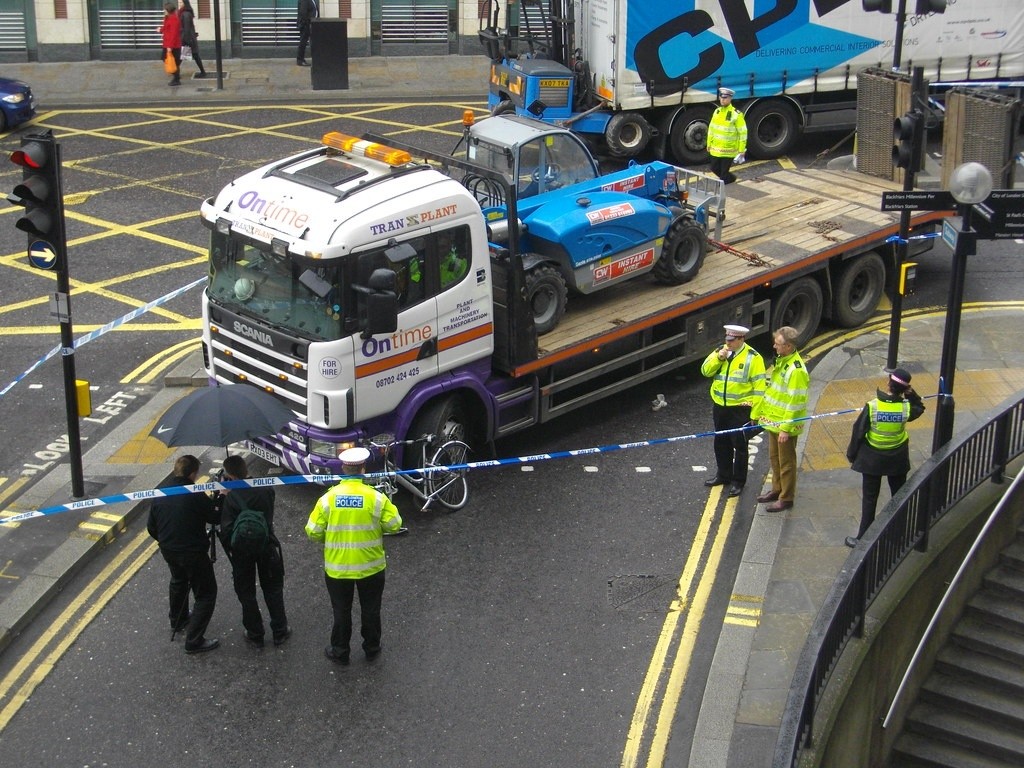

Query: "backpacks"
<box><xmin>229</xmin><ymin>489</ymin><xmax>269</xmax><ymax>555</ymax></box>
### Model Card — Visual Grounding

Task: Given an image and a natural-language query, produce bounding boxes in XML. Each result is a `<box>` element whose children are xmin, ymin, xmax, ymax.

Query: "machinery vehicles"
<box><xmin>467</xmin><ymin>115</ymin><xmax>727</xmax><ymax>338</ymax></box>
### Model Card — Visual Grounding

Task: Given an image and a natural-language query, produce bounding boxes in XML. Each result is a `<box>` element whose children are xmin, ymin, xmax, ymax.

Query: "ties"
<box><xmin>728</xmin><ymin>351</ymin><xmax>735</xmax><ymax>363</ymax></box>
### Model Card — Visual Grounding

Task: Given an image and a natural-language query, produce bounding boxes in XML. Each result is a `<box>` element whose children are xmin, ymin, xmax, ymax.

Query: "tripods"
<box><xmin>170</xmin><ymin>489</ymin><xmax>233</xmax><ymax>641</ymax></box>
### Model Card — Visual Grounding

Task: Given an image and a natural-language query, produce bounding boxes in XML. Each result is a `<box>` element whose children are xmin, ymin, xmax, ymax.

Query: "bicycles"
<box><xmin>358</xmin><ymin>433</ymin><xmax>473</xmax><ymax>514</ymax></box>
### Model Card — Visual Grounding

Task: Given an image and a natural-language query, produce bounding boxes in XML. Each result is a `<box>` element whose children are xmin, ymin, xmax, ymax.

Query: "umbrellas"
<box><xmin>149</xmin><ymin>381</ymin><xmax>299</xmax><ymax>458</ymax></box>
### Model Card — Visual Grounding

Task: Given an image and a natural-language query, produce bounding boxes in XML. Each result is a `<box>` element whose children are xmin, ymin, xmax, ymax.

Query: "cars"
<box><xmin>0</xmin><ymin>77</ymin><xmax>36</xmax><ymax>132</ymax></box>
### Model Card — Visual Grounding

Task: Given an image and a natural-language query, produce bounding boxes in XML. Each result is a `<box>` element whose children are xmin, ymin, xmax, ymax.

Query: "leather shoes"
<box><xmin>175</xmin><ymin>610</ymin><xmax>193</xmax><ymax>628</ymax></box>
<box><xmin>756</xmin><ymin>491</ymin><xmax>779</xmax><ymax>501</ymax></box>
<box><xmin>766</xmin><ymin>500</ymin><xmax>793</xmax><ymax>512</ymax></box>
<box><xmin>185</xmin><ymin>638</ymin><xmax>218</xmax><ymax>653</ymax></box>
<box><xmin>274</xmin><ymin>625</ymin><xmax>291</xmax><ymax>644</ymax></box>
<box><xmin>244</xmin><ymin>629</ymin><xmax>264</xmax><ymax>648</ymax></box>
<box><xmin>845</xmin><ymin>536</ymin><xmax>857</xmax><ymax>546</ymax></box>
<box><xmin>729</xmin><ymin>486</ymin><xmax>742</xmax><ymax>496</ymax></box>
<box><xmin>705</xmin><ymin>476</ymin><xmax>728</xmax><ymax>485</ymax></box>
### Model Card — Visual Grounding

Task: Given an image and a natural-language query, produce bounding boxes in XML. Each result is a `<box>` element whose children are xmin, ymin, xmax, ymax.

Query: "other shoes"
<box><xmin>298</xmin><ymin>60</ymin><xmax>311</xmax><ymax>66</ymax></box>
<box><xmin>196</xmin><ymin>73</ymin><xmax>206</xmax><ymax>77</ymax></box>
<box><xmin>366</xmin><ymin>641</ymin><xmax>382</xmax><ymax>659</ymax></box>
<box><xmin>168</xmin><ymin>81</ymin><xmax>181</xmax><ymax>86</ymax></box>
<box><xmin>325</xmin><ymin>646</ymin><xmax>348</xmax><ymax>665</ymax></box>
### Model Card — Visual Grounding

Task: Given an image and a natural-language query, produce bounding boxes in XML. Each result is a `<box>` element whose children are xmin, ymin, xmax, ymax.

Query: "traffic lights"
<box><xmin>8</xmin><ymin>128</ymin><xmax>65</xmax><ymax>273</ymax></box>
<box><xmin>889</xmin><ymin>110</ymin><xmax>923</xmax><ymax>175</ymax></box>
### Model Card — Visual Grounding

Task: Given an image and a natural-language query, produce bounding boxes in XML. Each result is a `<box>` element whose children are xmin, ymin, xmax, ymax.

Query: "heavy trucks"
<box><xmin>197</xmin><ymin>131</ymin><xmax>955</xmax><ymax>494</ymax></box>
<box><xmin>477</xmin><ymin>0</ymin><xmax>1024</xmax><ymax>161</ymax></box>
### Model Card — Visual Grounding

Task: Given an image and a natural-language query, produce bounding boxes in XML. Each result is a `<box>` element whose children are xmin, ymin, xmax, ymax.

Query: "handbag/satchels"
<box><xmin>180</xmin><ymin>44</ymin><xmax>192</xmax><ymax>61</ymax></box>
<box><xmin>165</xmin><ymin>51</ymin><xmax>177</xmax><ymax>73</ymax></box>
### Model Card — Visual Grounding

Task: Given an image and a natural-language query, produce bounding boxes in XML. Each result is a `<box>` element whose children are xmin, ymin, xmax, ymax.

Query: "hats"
<box><xmin>338</xmin><ymin>447</ymin><xmax>370</xmax><ymax>465</ymax></box>
<box><xmin>722</xmin><ymin>324</ymin><xmax>749</xmax><ymax>341</ymax></box>
<box><xmin>889</xmin><ymin>369</ymin><xmax>912</xmax><ymax>389</ymax></box>
<box><xmin>719</xmin><ymin>88</ymin><xmax>735</xmax><ymax>98</ymax></box>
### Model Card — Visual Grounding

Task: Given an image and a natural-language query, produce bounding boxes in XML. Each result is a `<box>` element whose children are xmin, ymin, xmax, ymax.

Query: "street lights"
<box><xmin>930</xmin><ymin>161</ymin><xmax>993</xmax><ymax>509</ymax></box>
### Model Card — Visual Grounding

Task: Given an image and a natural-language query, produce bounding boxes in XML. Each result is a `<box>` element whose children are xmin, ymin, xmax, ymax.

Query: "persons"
<box><xmin>220</xmin><ymin>455</ymin><xmax>290</xmax><ymax>641</ymax></box>
<box><xmin>296</xmin><ymin>0</ymin><xmax>320</xmax><ymax>65</ymax></box>
<box><xmin>409</xmin><ymin>236</ymin><xmax>467</xmax><ymax>289</ymax></box>
<box><xmin>707</xmin><ymin>87</ymin><xmax>748</xmax><ymax>184</ymax></box>
<box><xmin>750</xmin><ymin>327</ymin><xmax>809</xmax><ymax>512</ymax></box>
<box><xmin>305</xmin><ymin>447</ymin><xmax>402</xmax><ymax>664</ymax></box>
<box><xmin>701</xmin><ymin>324</ymin><xmax>767</xmax><ymax>496</ymax></box>
<box><xmin>157</xmin><ymin>0</ymin><xmax>206</xmax><ymax>86</ymax></box>
<box><xmin>845</xmin><ymin>368</ymin><xmax>926</xmax><ymax>549</ymax></box>
<box><xmin>147</xmin><ymin>454</ymin><xmax>230</xmax><ymax>654</ymax></box>
